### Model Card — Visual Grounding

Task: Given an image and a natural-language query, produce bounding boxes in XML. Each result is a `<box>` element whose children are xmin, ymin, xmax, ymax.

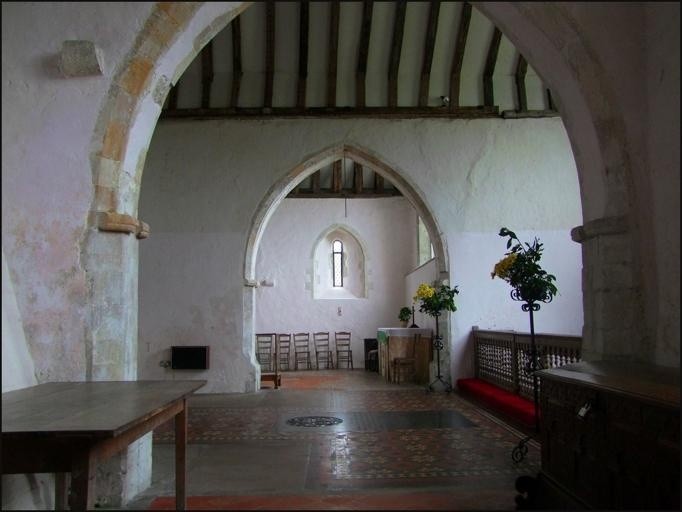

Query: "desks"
<box><xmin>534</xmin><ymin>359</ymin><xmax>682</xmax><ymax>511</ymax></box>
<box><xmin>377</xmin><ymin>327</ymin><xmax>432</xmax><ymax>384</ymax></box>
<box><xmin>0</xmin><ymin>379</ymin><xmax>206</xmax><ymax>512</ymax></box>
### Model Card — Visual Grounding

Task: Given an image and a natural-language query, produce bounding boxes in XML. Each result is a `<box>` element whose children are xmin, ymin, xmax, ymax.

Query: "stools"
<box><xmin>393</xmin><ymin>357</ymin><xmax>420</xmax><ymax>385</ymax></box>
<box><xmin>368</xmin><ymin>349</ymin><xmax>378</xmax><ymax>371</ymax></box>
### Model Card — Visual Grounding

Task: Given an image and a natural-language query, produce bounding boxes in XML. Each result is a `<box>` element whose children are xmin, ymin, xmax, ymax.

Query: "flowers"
<box><xmin>492</xmin><ymin>227</ymin><xmax>558</xmax><ymax>305</ymax></box>
<box><xmin>413</xmin><ymin>283</ymin><xmax>459</xmax><ymax>314</ymax></box>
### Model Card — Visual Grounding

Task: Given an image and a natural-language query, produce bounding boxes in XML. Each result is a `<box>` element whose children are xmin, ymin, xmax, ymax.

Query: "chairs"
<box><xmin>255</xmin><ymin>333</ymin><xmax>271</xmax><ymax>372</ymax></box>
<box><xmin>313</xmin><ymin>332</ymin><xmax>334</xmax><ymax>370</ymax></box>
<box><xmin>335</xmin><ymin>332</ymin><xmax>353</xmax><ymax>370</ymax></box>
<box><xmin>272</xmin><ymin>333</ymin><xmax>292</xmax><ymax>371</ymax></box>
<box><xmin>293</xmin><ymin>332</ymin><xmax>312</xmax><ymax>370</ymax></box>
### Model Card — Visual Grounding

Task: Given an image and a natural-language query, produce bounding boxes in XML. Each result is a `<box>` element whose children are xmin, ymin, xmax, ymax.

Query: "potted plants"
<box><xmin>398</xmin><ymin>307</ymin><xmax>412</xmax><ymax>328</ymax></box>
